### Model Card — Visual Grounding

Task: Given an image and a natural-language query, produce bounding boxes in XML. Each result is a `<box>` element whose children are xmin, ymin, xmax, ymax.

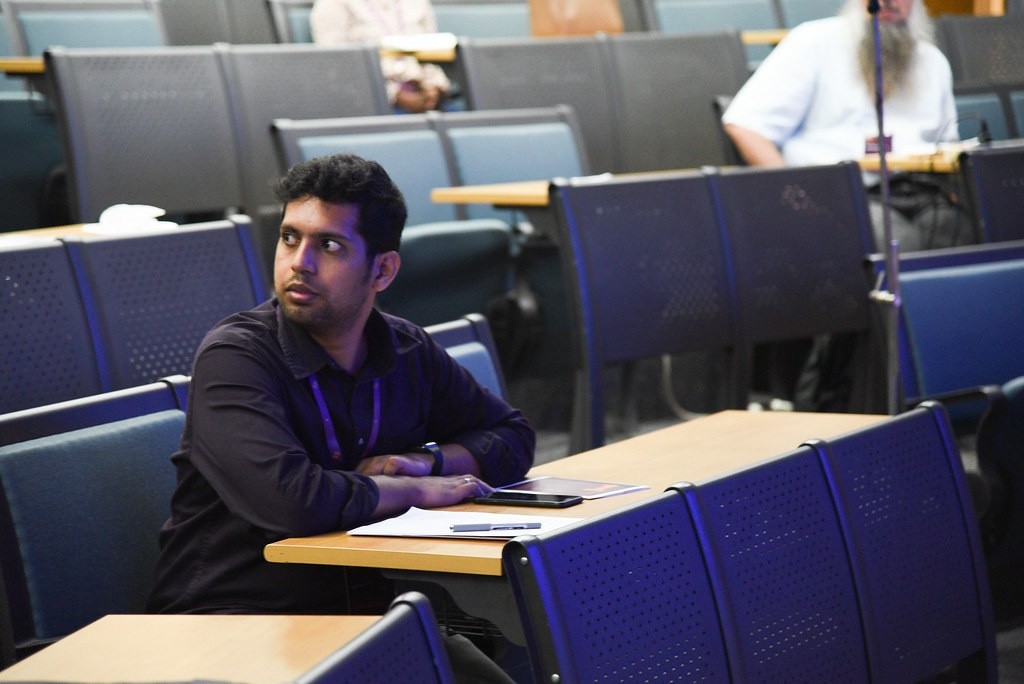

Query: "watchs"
<box><xmin>413</xmin><ymin>442</ymin><xmax>443</xmax><ymax>477</ymax></box>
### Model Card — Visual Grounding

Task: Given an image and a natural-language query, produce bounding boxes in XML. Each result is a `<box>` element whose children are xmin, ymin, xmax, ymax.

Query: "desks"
<box><xmin>1</xmin><ymin>55</ymin><xmax>68</xmax><ymax>215</ymax></box>
<box><xmin>431</xmin><ymin>164</ymin><xmax>718</xmax><ymax>438</ymax></box>
<box><xmin>742</xmin><ymin>27</ymin><xmax>795</xmax><ymax>45</ymax></box>
<box><xmin>0</xmin><ymin>614</ymin><xmax>383</xmax><ymax>683</ymax></box>
<box><xmin>263</xmin><ymin>408</ymin><xmax>893</xmax><ymax>683</ymax></box>
<box><xmin>862</xmin><ymin>148</ymin><xmax>984</xmax><ymax>246</ymax></box>
<box><xmin>388</xmin><ymin>37</ymin><xmax>465</xmax><ymax>87</ymax></box>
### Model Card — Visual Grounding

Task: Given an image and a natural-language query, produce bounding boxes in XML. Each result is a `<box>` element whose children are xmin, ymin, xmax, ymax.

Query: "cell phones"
<box><xmin>474</xmin><ymin>492</ymin><xmax>583</xmax><ymax>508</ymax></box>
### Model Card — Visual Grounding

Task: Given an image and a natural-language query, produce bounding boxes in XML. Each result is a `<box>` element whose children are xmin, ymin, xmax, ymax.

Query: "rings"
<box><xmin>464</xmin><ymin>478</ymin><xmax>470</xmax><ymax>482</ymax></box>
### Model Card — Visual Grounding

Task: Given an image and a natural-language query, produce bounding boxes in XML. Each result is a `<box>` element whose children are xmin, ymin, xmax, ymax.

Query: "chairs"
<box><xmin>0</xmin><ymin>0</ymin><xmax>1024</xmax><ymax>683</ymax></box>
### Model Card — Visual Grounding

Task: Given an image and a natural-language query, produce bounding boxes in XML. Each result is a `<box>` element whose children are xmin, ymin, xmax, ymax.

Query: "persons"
<box><xmin>308</xmin><ymin>0</ymin><xmax>469</xmax><ymax>115</ymax></box>
<box><xmin>719</xmin><ymin>0</ymin><xmax>977</xmax><ymax>255</ymax></box>
<box><xmin>154</xmin><ymin>154</ymin><xmax>537</xmax><ymax>684</ymax></box>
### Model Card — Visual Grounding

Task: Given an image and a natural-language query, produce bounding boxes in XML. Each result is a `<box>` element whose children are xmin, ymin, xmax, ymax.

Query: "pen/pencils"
<box><xmin>450</xmin><ymin>523</ymin><xmax>541</xmax><ymax>532</ymax></box>
